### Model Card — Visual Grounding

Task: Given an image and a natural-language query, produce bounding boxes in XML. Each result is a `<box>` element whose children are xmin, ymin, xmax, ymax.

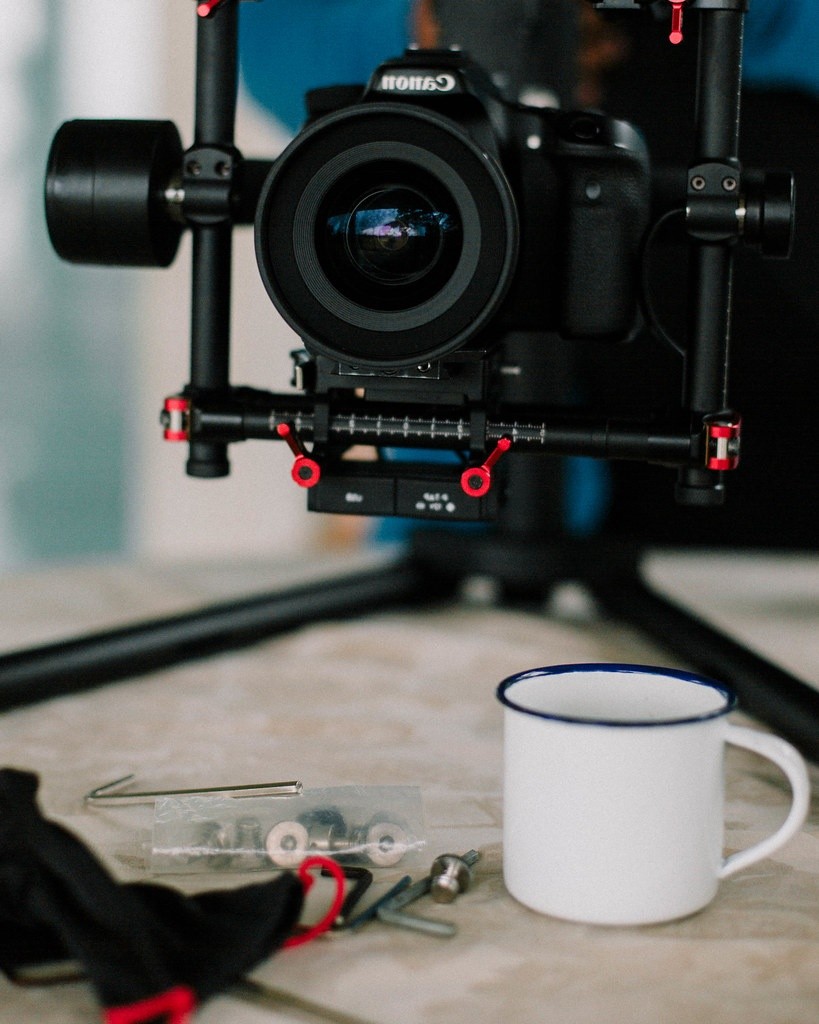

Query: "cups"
<box><xmin>495</xmin><ymin>661</ymin><xmax>812</xmax><ymax>929</ymax></box>
